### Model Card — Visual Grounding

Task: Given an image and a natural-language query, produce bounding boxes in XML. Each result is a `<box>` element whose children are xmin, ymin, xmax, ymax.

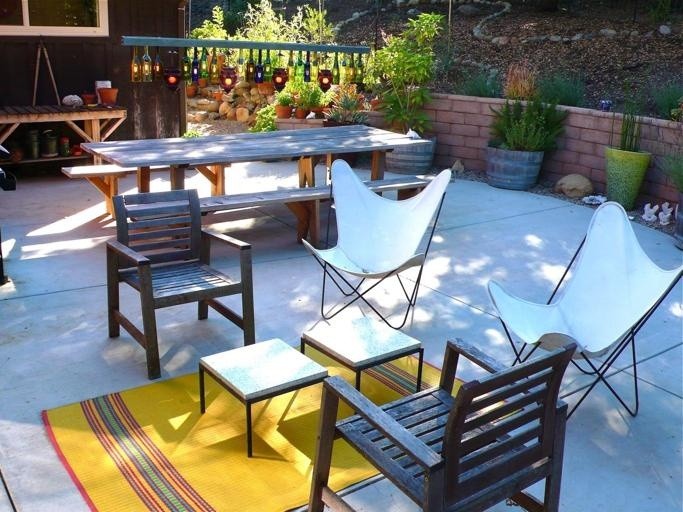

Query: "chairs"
<box><xmin>300</xmin><ymin>159</ymin><xmax>451</xmax><ymax>330</ymax></box>
<box><xmin>106</xmin><ymin>190</ymin><xmax>255</xmax><ymax>379</ymax></box>
<box><xmin>487</xmin><ymin>201</ymin><xmax>682</xmax><ymax>419</ymax></box>
<box><xmin>309</xmin><ymin>333</ymin><xmax>576</xmax><ymax>512</ymax></box>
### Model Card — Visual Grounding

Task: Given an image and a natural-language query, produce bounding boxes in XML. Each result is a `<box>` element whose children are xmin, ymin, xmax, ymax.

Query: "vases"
<box><xmin>99</xmin><ymin>88</ymin><xmax>118</xmax><ymax>106</ymax></box>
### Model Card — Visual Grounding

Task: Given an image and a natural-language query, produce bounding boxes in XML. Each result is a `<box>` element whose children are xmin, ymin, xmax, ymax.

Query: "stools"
<box><xmin>300</xmin><ymin>324</ymin><xmax>424</xmax><ymax>414</ymax></box>
<box><xmin>199</xmin><ymin>339</ymin><xmax>330</xmax><ymax>457</ymax></box>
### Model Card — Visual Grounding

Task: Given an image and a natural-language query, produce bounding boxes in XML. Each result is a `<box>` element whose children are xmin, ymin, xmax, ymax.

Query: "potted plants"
<box><xmin>482</xmin><ymin>97</ymin><xmax>570</xmax><ymax>190</ymax></box>
<box><xmin>654</xmin><ymin>153</ymin><xmax>683</xmax><ymax>251</ymax></box>
<box><xmin>604</xmin><ymin>82</ymin><xmax>651</xmax><ymax>212</ymax></box>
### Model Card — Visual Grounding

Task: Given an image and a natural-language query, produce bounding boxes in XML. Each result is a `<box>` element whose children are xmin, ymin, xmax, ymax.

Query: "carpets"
<box><xmin>41</xmin><ymin>344</ymin><xmax>524</xmax><ymax>512</ymax></box>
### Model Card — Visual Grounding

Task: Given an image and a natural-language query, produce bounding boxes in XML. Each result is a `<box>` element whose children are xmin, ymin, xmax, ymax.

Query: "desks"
<box><xmin>80</xmin><ymin>124</ymin><xmax>433</xmax><ymax>193</ymax></box>
<box><xmin>0</xmin><ymin>106</ymin><xmax>129</xmax><ymax>164</ymax></box>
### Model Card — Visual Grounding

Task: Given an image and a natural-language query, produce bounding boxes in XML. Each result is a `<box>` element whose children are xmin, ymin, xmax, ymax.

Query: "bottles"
<box><xmin>128</xmin><ymin>45</ymin><xmax>363</xmax><ymax>85</ymax></box>
<box><xmin>29</xmin><ymin>134</ymin><xmax>69</xmax><ymax>159</ymax></box>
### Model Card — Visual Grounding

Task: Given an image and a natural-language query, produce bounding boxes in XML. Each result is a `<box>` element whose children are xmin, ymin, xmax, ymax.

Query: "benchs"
<box><xmin>61</xmin><ymin>165</ymin><xmax>231</xmax><ymax>220</ymax></box>
<box><xmin>129</xmin><ymin>176</ymin><xmax>436</xmax><ymax>244</ymax></box>
